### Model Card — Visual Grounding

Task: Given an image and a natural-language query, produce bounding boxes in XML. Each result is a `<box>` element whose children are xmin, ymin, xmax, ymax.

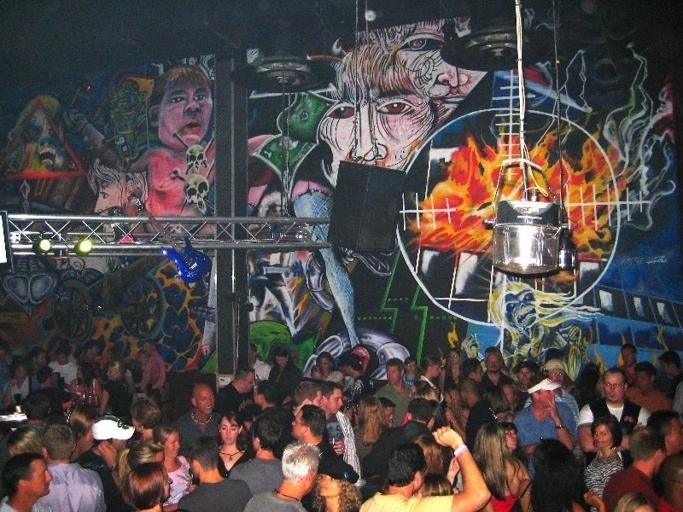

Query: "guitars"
<box><xmin>128</xmin><ymin>194</ymin><xmax>212</xmax><ymax>283</ymax></box>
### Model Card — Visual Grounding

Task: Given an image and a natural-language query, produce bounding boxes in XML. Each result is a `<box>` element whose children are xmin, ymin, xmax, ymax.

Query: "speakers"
<box><xmin>328</xmin><ymin>160</ymin><xmax>406</xmax><ymax>256</ymax></box>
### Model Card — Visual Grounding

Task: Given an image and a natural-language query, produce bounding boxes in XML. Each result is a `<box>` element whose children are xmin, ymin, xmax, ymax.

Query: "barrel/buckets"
<box><xmin>491</xmin><ymin>160</ymin><xmax>567</xmax><ymax>276</ymax></box>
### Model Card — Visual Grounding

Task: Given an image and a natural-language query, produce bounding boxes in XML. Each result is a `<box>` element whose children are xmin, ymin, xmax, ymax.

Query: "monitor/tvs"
<box><xmin>0</xmin><ymin>211</ymin><xmax>12</xmax><ymax>263</ymax></box>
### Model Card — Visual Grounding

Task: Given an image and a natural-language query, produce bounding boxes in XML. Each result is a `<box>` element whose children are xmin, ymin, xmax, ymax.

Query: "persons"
<box><xmin>61</xmin><ymin>65</ymin><xmax>216</xmax><ymax>236</ymax></box>
<box><xmin>1</xmin><ymin>337</ymin><xmax>682</xmax><ymax>512</ymax></box>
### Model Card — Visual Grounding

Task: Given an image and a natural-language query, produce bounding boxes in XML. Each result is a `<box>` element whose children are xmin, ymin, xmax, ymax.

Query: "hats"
<box><xmin>527</xmin><ymin>377</ymin><xmax>561</xmax><ymax>394</ymax></box>
<box><xmin>319</xmin><ymin>458</ymin><xmax>359</xmax><ymax>485</ymax></box>
<box><xmin>91</xmin><ymin>415</ymin><xmax>136</xmax><ymax>441</ymax></box>
<box><xmin>543</xmin><ymin>360</ymin><xmax>564</xmax><ymax>371</ymax></box>
